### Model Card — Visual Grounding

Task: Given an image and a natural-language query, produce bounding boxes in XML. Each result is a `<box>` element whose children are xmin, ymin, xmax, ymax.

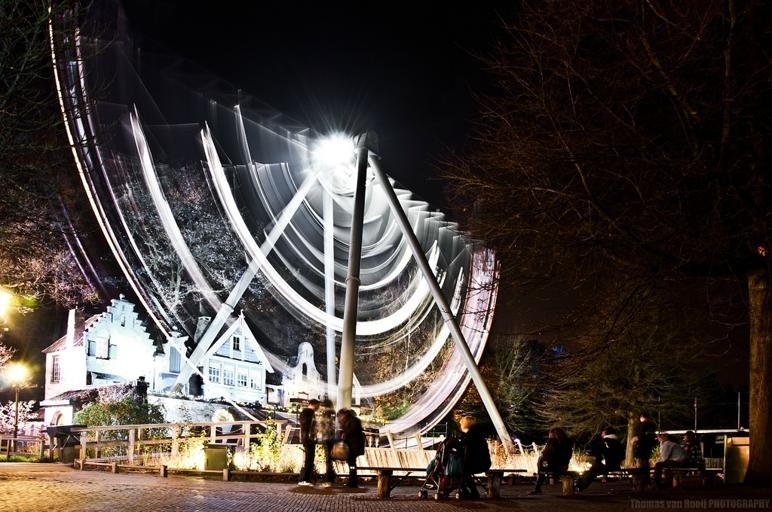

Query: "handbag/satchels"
<box><xmin>328</xmin><ymin>440</ymin><xmax>350</xmax><ymax>461</ymax></box>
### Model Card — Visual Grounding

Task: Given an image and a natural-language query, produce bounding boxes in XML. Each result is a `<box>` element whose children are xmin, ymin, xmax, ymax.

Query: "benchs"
<box><xmin>543</xmin><ymin>465</ymin><xmax>726</xmax><ymax>498</ymax></box>
<box><xmin>350</xmin><ymin>463</ymin><xmax>526</xmax><ymax>502</ymax></box>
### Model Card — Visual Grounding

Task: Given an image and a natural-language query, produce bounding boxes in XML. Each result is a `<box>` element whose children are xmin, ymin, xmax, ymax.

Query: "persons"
<box><xmin>651</xmin><ymin>434</ymin><xmax>684</xmax><ymax>490</ymax></box>
<box><xmin>337</xmin><ymin>408</ymin><xmax>366</xmax><ymax>488</ymax></box>
<box><xmin>633</xmin><ymin>413</ymin><xmax>657</xmax><ymax>488</ymax></box>
<box><xmin>577</xmin><ymin>428</ymin><xmax>626</xmax><ymax>492</ymax></box>
<box><xmin>316</xmin><ymin>399</ymin><xmax>336</xmax><ymax>482</ymax></box>
<box><xmin>528</xmin><ymin>427</ymin><xmax>572</xmax><ymax>495</ymax></box>
<box><xmin>299</xmin><ymin>399</ymin><xmax>319</xmax><ymax>484</ymax></box>
<box><xmin>672</xmin><ymin>431</ymin><xmax>702</xmax><ymax>488</ymax></box>
<box><xmin>452</xmin><ymin>416</ymin><xmax>492</xmax><ymax>501</ymax></box>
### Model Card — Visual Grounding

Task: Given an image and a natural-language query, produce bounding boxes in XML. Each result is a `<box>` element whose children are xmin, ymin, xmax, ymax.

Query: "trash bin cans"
<box><xmin>200</xmin><ymin>442</ymin><xmax>238</xmax><ymax>479</ymax></box>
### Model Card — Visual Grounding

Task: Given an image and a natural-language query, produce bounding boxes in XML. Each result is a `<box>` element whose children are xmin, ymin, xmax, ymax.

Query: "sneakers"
<box><xmin>316</xmin><ymin>483</ymin><xmax>331</xmax><ymax>490</ymax></box>
<box><xmin>297</xmin><ymin>481</ymin><xmax>315</xmax><ymax>487</ymax></box>
